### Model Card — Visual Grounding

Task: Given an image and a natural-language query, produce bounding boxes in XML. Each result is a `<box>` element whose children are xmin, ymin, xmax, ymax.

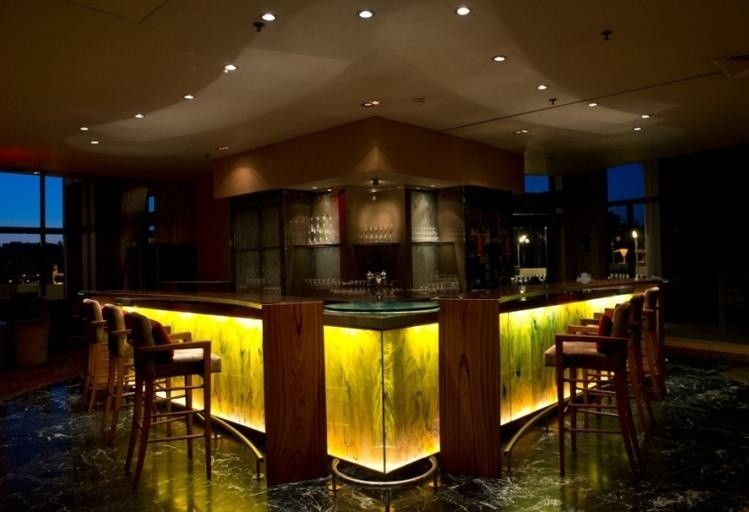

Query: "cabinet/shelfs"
<box><xmin>225</xmin><ymin>184</ymin><xmax>517</xmax><ymax>302</ymax></box>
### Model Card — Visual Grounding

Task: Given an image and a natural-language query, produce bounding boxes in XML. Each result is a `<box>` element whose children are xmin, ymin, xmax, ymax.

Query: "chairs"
<box><xmin>544</xmin><ymin>286</ymin><xmax>666</xmax><ymax>479</ymax></box>
<box><xmin>80</xmin><ymin>298</ymin><xmax>221</xmax><ymax>491</ymax></box>
